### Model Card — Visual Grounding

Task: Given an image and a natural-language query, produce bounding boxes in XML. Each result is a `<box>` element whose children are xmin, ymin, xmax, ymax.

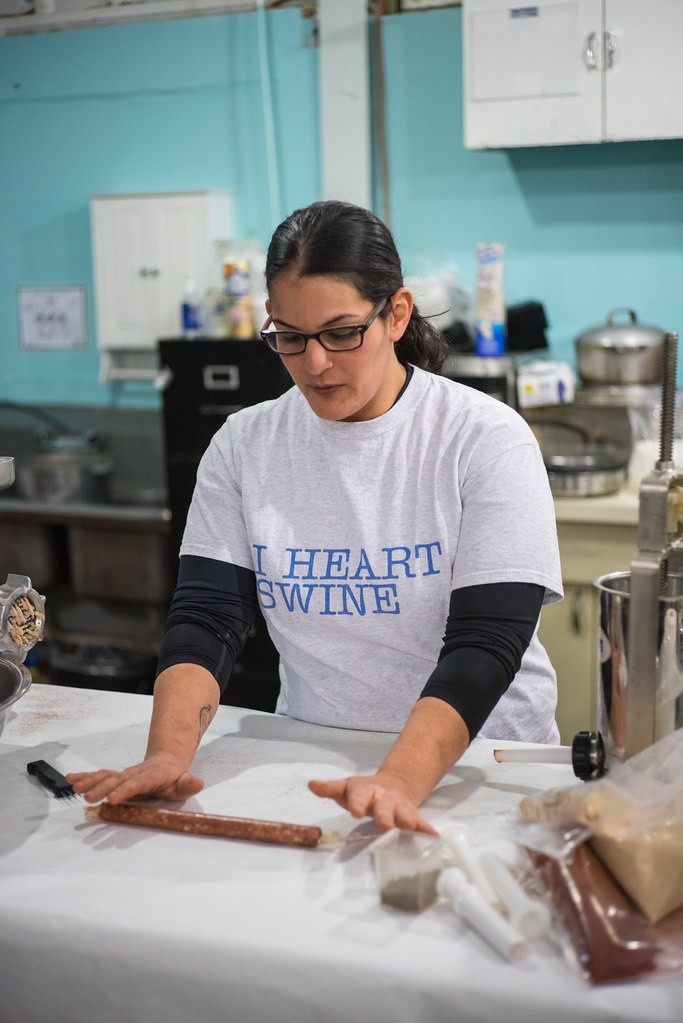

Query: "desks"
<box><xmin>1</xmin><ymin>685</ymin><xmax>683</xmax><ymax>1022</ymax></box>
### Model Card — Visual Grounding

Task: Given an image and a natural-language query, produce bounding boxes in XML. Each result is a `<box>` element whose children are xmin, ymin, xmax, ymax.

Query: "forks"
<box><xmin>27</xmin><ymin>759</ymin><xmax>82</xmax><ymax>801</ymax></box>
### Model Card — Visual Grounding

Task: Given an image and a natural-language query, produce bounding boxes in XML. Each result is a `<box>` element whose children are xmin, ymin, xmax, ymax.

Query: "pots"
<box><xmin>591</xmin><ymin>567</ymin><xmax>683</xmax><ymax>759</ymax></box>
<box><xmin>546</xmin><ymin>450</ymin><xmax>627</xmax><ymax>497</ymax></box>
<box><xmin>18</xmin><ymin>454</ymin><xmax>113</xmax><ymax>505</ymax></box>
<box><xmin>574</xmin><ymin>308</ymin><xmax>665</xmax><ymax>387</ymax></box>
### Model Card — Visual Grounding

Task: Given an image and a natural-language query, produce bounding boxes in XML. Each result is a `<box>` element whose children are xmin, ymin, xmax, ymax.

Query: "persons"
<box><xmin>61</xmin><ymin>200</ymin><xmax>562</xmax><ymax>838</ymax></box>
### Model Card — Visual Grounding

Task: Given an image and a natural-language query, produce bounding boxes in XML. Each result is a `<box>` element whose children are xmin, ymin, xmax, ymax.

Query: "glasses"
<box><xmin>261</xmin><ymin>291</ymin><xmax>393</xmax><ymax>355</ymax></box>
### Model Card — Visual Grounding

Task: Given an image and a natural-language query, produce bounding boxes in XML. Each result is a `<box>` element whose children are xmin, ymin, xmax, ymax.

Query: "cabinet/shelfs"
<box><xmin>536</xmin><ymin>521</ymin><xmax>638</xmax><ymax>747</ymax></box>
<box><xmin>89</xmin><ymin>192</ymin><xmax>238</xmax><ymax>384</ymax></box>
<box><xmin>461</xmin><ymin>0</ymin><xmax>683</xmax><ymax>149</ymax></box>
